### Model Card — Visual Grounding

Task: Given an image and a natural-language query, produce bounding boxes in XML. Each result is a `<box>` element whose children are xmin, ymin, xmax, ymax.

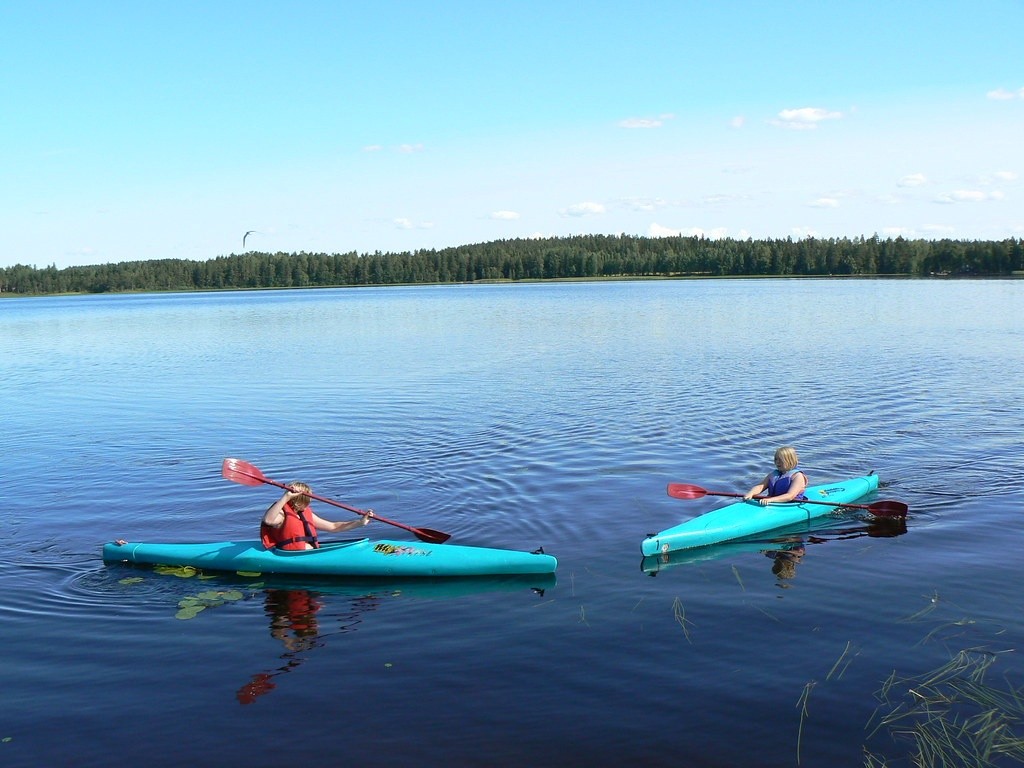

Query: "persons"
<box><xmin>763</xmin><ymin>538</ymin><xmax>805</xmax><ymax>582</ymax></box>
<box><xmin>259</xmin><ymin>482</ymin><xmax>372</xmax><ymax>550</ymax></box>
<box><xmin>263</xmin><ymin>589</ymin><xmax>320</xmax><ymax>650</ymax></box>
<box><xmin>743</xmin><ymin>447</ymin><xmax>809</xmax><ymax>505</ymax></box>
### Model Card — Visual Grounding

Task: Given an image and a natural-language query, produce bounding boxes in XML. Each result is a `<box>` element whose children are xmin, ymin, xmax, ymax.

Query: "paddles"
<box><xmin>221</xmin><ymin>459</ymin><xmax>451</xmax><ymax>544</ymax></box>
<box><xmin>667</xmin><ymin>482</ymin><xmax>908</xmax><ymax>516</ymax></box>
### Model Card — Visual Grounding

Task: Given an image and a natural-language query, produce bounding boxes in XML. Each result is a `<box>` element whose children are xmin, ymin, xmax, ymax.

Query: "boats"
<box><xmin>639</xmin><ymin>469</ymin><xmax>884</xmax><ymax>560</ymax></box>
<box><xmin>101</xmin><ymin>533</ymin><xmax>558</xmax><ymax>580</ymax></box>
